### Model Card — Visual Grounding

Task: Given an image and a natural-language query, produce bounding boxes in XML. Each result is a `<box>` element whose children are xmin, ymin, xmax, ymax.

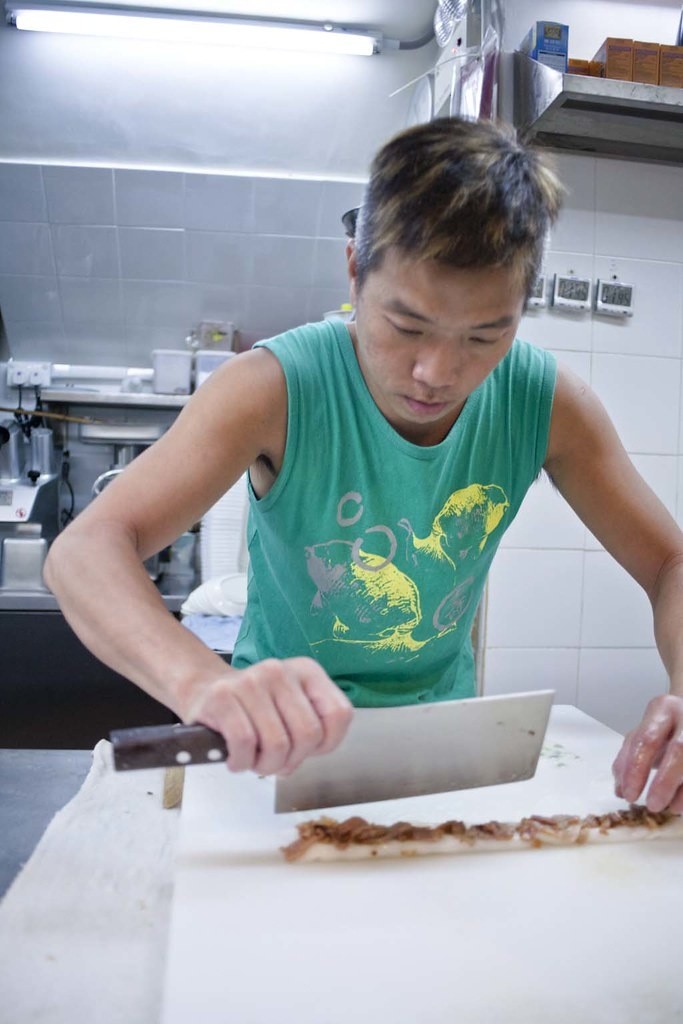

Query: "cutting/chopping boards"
<box><xmin>166</xmin><ymin>704</ymin><xmax>683</xmax><ymax>1024</ymax></box>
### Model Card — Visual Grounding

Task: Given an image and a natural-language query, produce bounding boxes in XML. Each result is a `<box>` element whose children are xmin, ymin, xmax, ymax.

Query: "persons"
<box><xmin>41</xmin><ymin>118</ymin><xmax>683</xmax><ymax>818</ymax></box>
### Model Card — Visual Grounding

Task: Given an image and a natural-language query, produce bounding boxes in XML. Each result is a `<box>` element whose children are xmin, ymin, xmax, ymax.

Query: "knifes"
<box><xmin>111</xmin><ymin>688</ymin><xmax>555</xmax><ymax>814</ymax></box>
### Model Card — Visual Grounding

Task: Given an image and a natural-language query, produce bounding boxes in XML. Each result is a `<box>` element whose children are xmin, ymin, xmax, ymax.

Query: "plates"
<box><xmin>201</xmin><ymin>469</ymin><xmax>250</xmax><ymax>584</ymax></box>
<box><xmin>181</xmin><ymin>572</ymin><xmax>248</xmax><ymax>618</ymax></box>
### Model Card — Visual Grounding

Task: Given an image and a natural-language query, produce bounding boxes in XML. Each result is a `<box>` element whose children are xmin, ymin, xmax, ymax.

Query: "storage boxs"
<box><xmin>518</xmin><ymin>20</ymin><xmax>569</xmax><ymax>73</ymax></box>
<box><xmin>659</xmin><ymin>44</ymin><xmax>683</xmax><ymax>88</ymax></box>
<box><xmin>150</xmin><ymin>347</ymin><xmax>194</xmax><ymax>394</ymax></box>
<box><xmin>194</xmin><ymin>350</ymin><xmax>236</xmax><ymax>389</ymax></box>
<box><xmin>591</xmin><ymin>37</ymin><xmax>633</xmax><ymax>82</ymax></box>
<box><xmin>568</xmin><ymin>59</ymin><xmax>589</xmax><ymax>75</ymax></box>
<box><xmin>633</xmin><ymin>41</ymin><xmax>660</xmax><ymax>85</ymax></box>
